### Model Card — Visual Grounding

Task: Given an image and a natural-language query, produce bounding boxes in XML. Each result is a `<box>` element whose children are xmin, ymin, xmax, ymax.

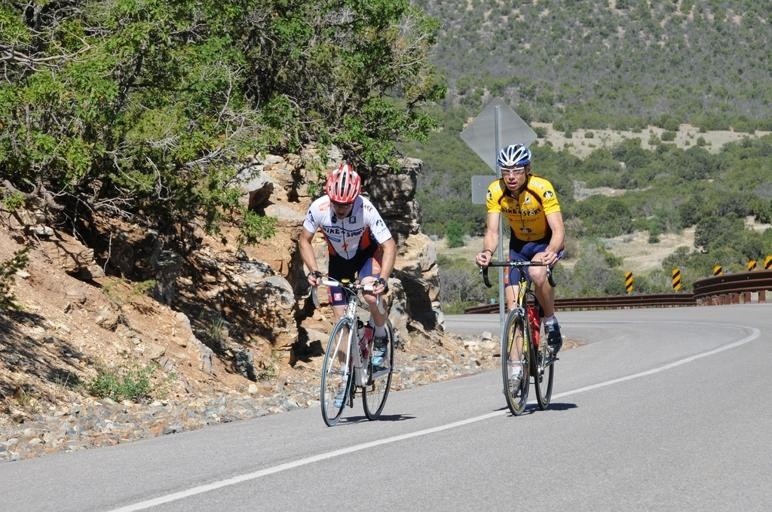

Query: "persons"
<box><xmin>472</xmin><ymin>142</ymin><xmax>567</xmax><ymax>399</ymax></box>
<box><xmin>296</xmin><ymin>163</ymin><xmax>397</xmax><ymax>410</ymax></box>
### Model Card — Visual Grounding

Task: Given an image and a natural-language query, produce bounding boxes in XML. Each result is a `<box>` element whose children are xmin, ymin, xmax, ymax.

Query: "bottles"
<box><xmin>358</xmin><ymin>316</ymin><xmax>373</xmax><ymax>359</ymax></box>
<box><xmin>527</xmin><ymin>302</ymin><xmax>541</xmax><ymax>348</ymax></box>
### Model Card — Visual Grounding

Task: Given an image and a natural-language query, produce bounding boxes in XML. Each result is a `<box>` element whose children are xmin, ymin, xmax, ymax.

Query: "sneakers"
<box><xmin>540</xmin><ymin>320</ymin><xmax>562</xmax><ymax>361</ymax></box>
<box><xmin>335</xmin><ymin>379</ymin><xmax>351</xmax><ymax>410</ymax></box>
<box><xmin>511</xmin><ymin>366</ymin><xmax>523</xmax><ymax>394</ymax></box>
<box><xmin>370</xmin><ymin>326</ymin><xmax>390</xmax><ymax>367</ymax></box>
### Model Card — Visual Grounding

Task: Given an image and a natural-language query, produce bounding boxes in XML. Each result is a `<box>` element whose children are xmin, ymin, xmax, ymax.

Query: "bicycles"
<box><xmin>301</xmin><ymin>271</ymin><xmax>395</xmax><ymax>428</ymax></box>
<box><xmin>480</xmin><ymin>261</ymin><xmax>559</xmax><ymax>415</ymax></box>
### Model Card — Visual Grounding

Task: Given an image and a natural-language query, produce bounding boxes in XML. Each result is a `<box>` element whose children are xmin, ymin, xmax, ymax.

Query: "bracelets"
<box><xmin>481</xmin><ymin>248</ymin><xmax>494</xmax><ymax>257</ymax></box>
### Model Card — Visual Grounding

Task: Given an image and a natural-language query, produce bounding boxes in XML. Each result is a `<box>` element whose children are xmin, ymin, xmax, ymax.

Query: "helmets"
<box><xmin>324</xmin><ymin>165</ymin><xmax>361</xmax><ymax>206</ymax></box>
<box><xmin>495</xmin><ymin>143</ymin><xmax>531</xmax><ymax>170</ymax></box>
